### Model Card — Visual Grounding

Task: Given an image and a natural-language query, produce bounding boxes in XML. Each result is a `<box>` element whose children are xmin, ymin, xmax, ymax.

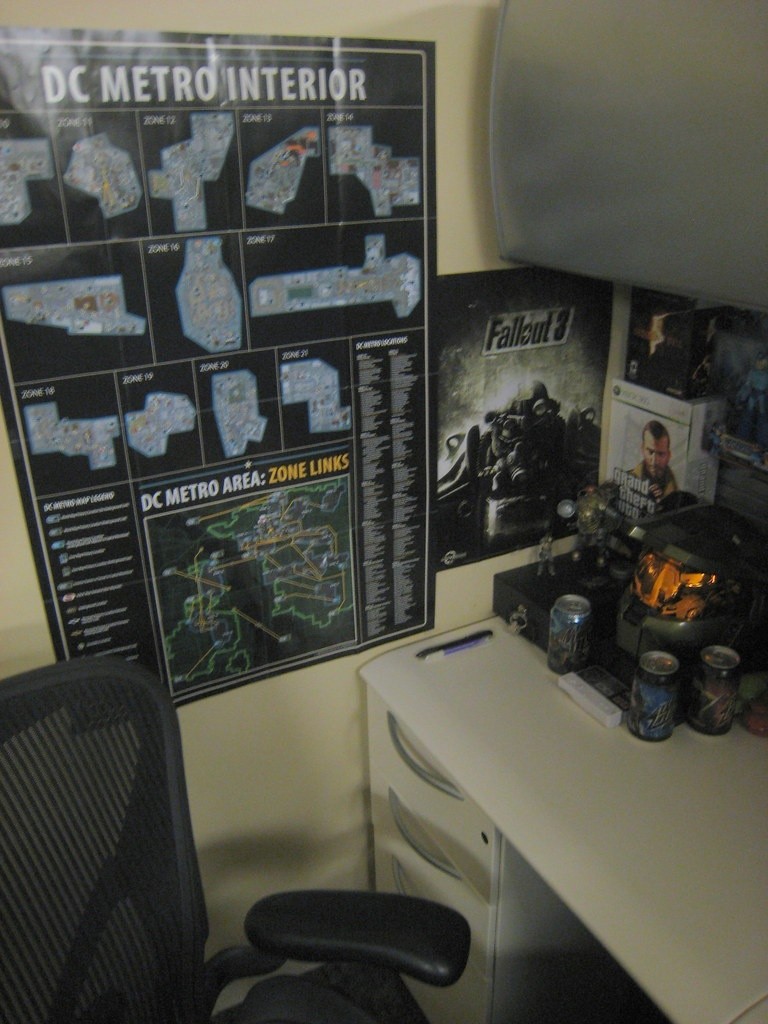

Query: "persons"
<box><xmin>626</xmin><ymin>419</ymin><xmax>676</xmax><ymax>506</ymax></box>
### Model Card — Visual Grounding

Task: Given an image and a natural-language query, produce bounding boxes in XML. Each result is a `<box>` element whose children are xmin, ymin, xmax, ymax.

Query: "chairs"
<box><xmin>0</xmin><ymin>659</ymin><xmax>471</xmax><ymax>1024</ymax></box>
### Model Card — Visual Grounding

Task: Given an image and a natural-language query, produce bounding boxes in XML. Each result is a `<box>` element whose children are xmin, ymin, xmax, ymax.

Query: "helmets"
<box><xmin>615</xmin><ymin>510</ymin><xmax>768</xmax><ymax>665</ymax></box>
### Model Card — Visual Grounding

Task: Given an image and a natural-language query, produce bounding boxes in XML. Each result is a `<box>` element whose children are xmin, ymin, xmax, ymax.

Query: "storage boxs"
<box><xmin>605</xmin><ymin>378</ymin><xmax>727</xmax><ymax>528</ymax></box>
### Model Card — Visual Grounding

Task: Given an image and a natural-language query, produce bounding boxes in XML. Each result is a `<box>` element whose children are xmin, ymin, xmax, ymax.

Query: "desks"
<box><xmin>358</xmin><ymin>614</ymin><xmax>768</xmax><ymax>1024</ymax></box>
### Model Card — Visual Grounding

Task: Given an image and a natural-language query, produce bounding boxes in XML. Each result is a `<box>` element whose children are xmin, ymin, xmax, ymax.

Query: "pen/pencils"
<box><xmin>416</xmin><ymin>629</ymin><xmax>494</xmax><ymax>663</ymax></box>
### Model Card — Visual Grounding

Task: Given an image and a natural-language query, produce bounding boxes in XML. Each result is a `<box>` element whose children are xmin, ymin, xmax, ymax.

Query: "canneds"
<box><xmin>627</xmin><ymin>651</ymin><xmax>680</xmax><ymax>742</ymax></box>
<box><xmin>686</xmin><ymin>645</ymin><xmax>740</xmax><ymax>736</ymax></box>
<box><xmin>546</xmin><ymin>594</ymin><xmax>591</xmax><ymax>674</ymax></box>
